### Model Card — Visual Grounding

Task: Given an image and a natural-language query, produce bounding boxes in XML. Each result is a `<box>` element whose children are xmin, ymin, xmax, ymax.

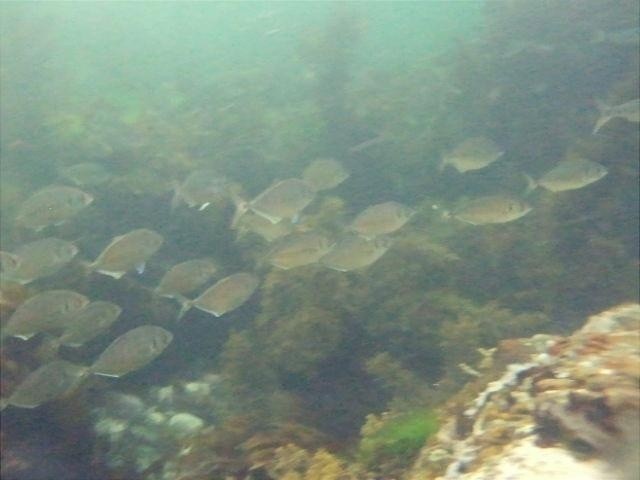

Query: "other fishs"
<box><xmin>0</xmin><ymin>95</ymin><xmax>640</xmax><ymax>415</ymax></box>
